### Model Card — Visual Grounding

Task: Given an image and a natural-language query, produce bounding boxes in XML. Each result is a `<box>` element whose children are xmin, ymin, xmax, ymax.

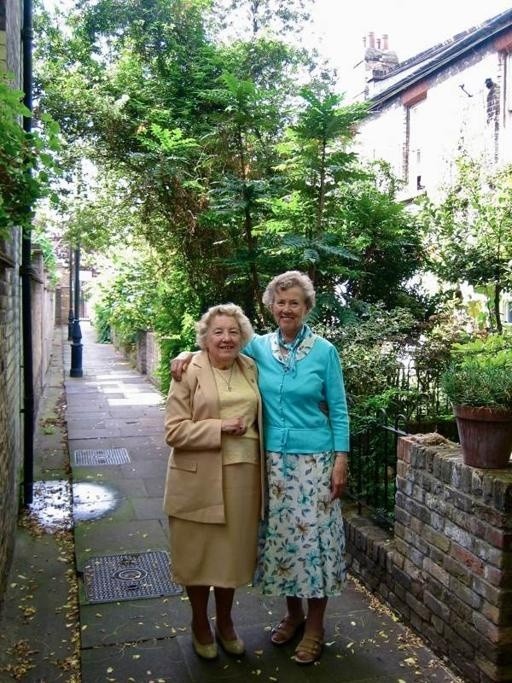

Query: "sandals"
<box><xmin>271</xmin><ymin>616</ymin><xmax>308</xmax><ymax>644</ymax></box>
<box><xmin>295</xmin><ymin>628</ymin><xmax>325</xmax><ymax>663</ymax></box>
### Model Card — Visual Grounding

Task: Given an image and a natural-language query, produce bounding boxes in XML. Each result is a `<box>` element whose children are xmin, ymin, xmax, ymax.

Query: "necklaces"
<box><xmin>215</xmin><ymin>363</ymin><xmax>233</xmax><ymax>392</ymax></box>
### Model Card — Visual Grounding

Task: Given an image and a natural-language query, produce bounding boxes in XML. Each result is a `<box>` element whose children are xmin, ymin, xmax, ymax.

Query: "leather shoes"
<box><xmin>214</xmin><ymin>617</ymin><xmax>244</xmax><ymax>654</ymax></box>
<box><xmin>191</xmin><ymin>624</ymin><xmax>218</xmax><ymax>659</ymax></box>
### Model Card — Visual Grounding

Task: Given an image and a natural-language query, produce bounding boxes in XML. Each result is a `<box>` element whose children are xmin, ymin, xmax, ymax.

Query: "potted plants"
<box><xmin>443</xmin><ymin>356</ymin><xmax>511</xmax><ymax>468</ymax></box>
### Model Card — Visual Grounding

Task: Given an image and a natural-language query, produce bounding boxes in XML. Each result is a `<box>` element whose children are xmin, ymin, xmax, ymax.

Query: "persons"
<box><xmin>168</xmin><ymin>269</ymin><xmax>353</xmax><ymax>664</ymax></box>
<box><xmin>161</xmin><ymin>302</ymin><xmax>266</xmax><ymax>660</ymax></box>
